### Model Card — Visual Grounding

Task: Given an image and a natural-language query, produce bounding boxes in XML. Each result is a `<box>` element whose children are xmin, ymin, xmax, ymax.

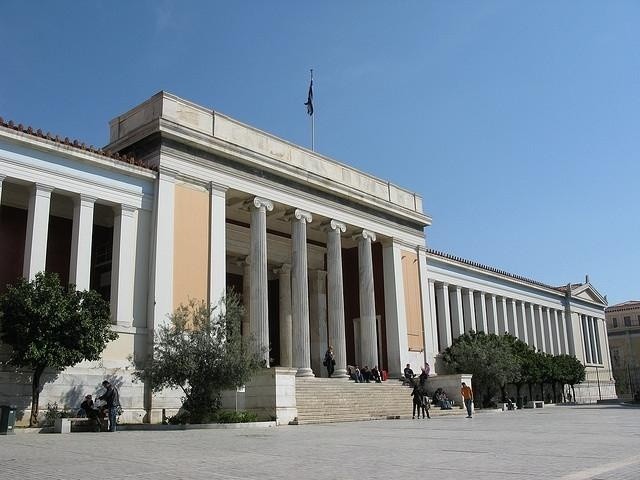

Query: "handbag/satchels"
<box><xmin>425</xmin><ymin>402</ymin><xmax>430</xmax><ymax>411</ymax></box>
<box><xmin>323</xmin><ymin>360</ymin><xmax>336</xmax><ymax>366</ymax></box>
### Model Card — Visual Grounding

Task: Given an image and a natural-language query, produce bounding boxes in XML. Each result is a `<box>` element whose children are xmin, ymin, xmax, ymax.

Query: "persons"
<box><xmin>404</xmin><ymin>364</ymin><xmax>418</xmax><ymax>389</ymax></box>
<box><xmin>94</xmin><ymin>381</ymin><xmax>120</xmax><ymax>432</ymax></box>
<box><xmin>360</xmin><ymin>365</ymin><xmax>372</xmax><ymax>383</ymax></box>
<box><xmin>460</xmin><ymin>382</ymin><xmax>474</xmax><ymax>418</ymax></box>
<box><xmin>410</xmin><ymin>384</ymin><xmax>423</xmax><ymax>419</ymax></box>
<box><xmin>324</xmin><ymin>345</ymin><xmax>336</xmax><ymax>377</ymax></box>
<box><xmin>80</xmin><ymin>394</ymin><xmax>105</xmax><ymax>432</ymax></box>
<box><xmin>419</xmin><ymin>362</ymin><xmax>430</xmax><ymax>385</ymax></box>
<box><xmin>419</xmin><ymin>387</ymin><xmax>433</xmax><ymax>419</ymax></box>
<box><xmin>433</xmin><ymin>390</ymin><xmax>452</xmax><ymax>409</ymax></box>
<box><xmin>348</xmin><ymin>365</ymin><xmax>365</xmax><ymax>383</ymax></box>
<box><xmin>371</xmin><ymin>366</ymin><xmax>382</xmax><ymax>383</ymax></box>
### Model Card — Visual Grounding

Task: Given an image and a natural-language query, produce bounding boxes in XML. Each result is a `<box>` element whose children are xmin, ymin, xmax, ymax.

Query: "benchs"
<box><xmin>53</xmin><ymin>416</ymin><xmax>121</xmax><ymax>434</ymax></box>
<box><xmin>502</xmin><ymin>402</ymin><xmax>517</xmax><ymax>411</ymax></box>
<box><xmin>531</xmin><ymin>400</ymin><xmax>544</xmax><ymax>409</ymax></box>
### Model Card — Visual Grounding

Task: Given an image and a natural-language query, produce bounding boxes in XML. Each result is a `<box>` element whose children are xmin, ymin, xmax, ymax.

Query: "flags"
<box><xmin>304</xmin><ymin>74</ymin><xmax>316</xmax><ymax>117</ymax></box>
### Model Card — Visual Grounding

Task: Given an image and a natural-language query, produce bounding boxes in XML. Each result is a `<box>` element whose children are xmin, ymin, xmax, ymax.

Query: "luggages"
<box><xmin>380</xmin><ymin>371</ymin><xmax>386</xmax><ymax>381</ymax></box>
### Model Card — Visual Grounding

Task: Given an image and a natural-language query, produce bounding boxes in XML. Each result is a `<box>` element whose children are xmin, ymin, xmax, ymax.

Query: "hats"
<box><xmin>85</xmin><ymin>395</ymin><xmax>92</xmax><ymax>399</ymax></box>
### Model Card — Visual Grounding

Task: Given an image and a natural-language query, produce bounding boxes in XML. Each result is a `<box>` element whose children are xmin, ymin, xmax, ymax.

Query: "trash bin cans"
<box><xmin>0</xmin><ymin>404</ymin><xmax>18</xmax><ymax>435</ymax></box>
